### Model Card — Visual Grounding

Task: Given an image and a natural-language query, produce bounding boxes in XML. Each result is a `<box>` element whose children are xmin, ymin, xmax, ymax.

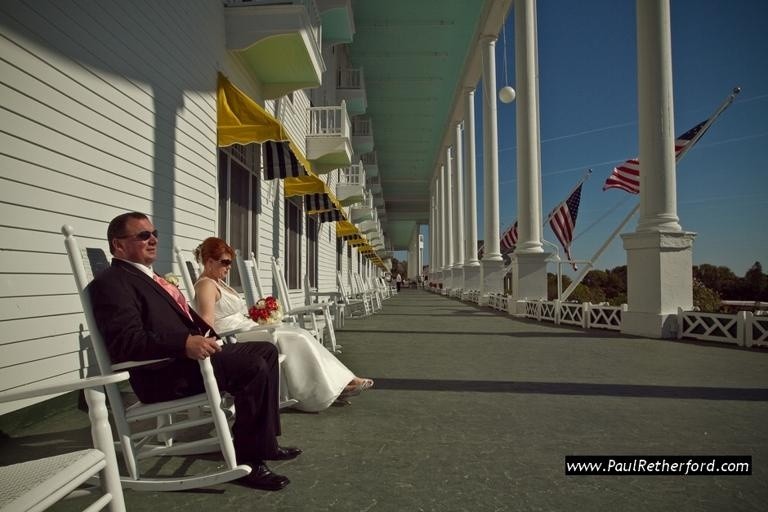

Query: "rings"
<box><xmin>204</xmin><ymin>351</ymin><xmax>207</xmax><ymax>357</ymax></box>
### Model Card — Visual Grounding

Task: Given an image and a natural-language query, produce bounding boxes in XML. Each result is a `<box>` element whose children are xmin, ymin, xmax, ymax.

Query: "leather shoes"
<box><xmin>232</xmin><ymin>461</ymin><xmax>290</xmax><ymax>491</ymax></box>
<box><xmin>267</xmin><ymin>446</ymin><xmax>302</xmax><ymax>459</ymax></box>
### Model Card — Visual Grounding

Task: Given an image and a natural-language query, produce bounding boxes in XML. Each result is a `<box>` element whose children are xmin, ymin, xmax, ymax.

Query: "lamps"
<box><xmin>499</xmin><ymin>15</ymin><xmax>515</xmax><ymax>104</ymax></box>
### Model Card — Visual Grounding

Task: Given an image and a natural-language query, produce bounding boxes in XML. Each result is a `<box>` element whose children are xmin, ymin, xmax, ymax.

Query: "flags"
<box><xmin>501</xmin><ymin>218</ymin><xmax>521</xmax><ymax>251</ymax></box>
<box><xmin>545</xmin><ymin>182</ymin><xmax>582</xmax><ymax>270</ymax></box>
<box><xmin>602</xmin><ymin>117</ymin><xmax>712</xmax><ymax>198</ymax></box>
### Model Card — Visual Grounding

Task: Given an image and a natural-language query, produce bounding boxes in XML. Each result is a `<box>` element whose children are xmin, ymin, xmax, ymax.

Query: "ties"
<box><xmin>153</xmin><ymin>273</ymin><xmax>191</xmax><ymax>319</ymax></box>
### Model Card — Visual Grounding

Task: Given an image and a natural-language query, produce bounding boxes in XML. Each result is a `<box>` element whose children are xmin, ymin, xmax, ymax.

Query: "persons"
<box><xmin>396</xmin><ymin>272</ymin><xmax>401</xmax><ymax>291</ymax></box>
<box><xmin>195</xmin><ymin>236</ymin><xmax>376</xmax><ymax>413</ymax></box>
<box><xmin>86</xmin><ymin>210</ymin><xmax>304</xmax><ymax>491</ymax></box>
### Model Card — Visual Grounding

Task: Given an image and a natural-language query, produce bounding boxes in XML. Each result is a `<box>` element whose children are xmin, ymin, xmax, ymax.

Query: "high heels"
<box><xmin>338</xmin><ymin>377</ymin><xmax>375</xmax><ymax>406</ymax></box>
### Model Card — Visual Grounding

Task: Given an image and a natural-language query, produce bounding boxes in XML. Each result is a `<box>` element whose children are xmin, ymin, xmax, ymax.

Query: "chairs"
<box><xmin>0</xmin><ymin>225</ymin><xmax>398</xmax><ymax>512</ymax></box>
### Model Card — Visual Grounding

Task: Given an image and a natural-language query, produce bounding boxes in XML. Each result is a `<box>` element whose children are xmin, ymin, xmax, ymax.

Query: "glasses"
<box><xmin>114</xmin><ymin>228</ymin><xmax>160</xmax><ymax>242</ymax></box>
<box><xmin>213</xmin><ymin>258</ymin><xmax>233</xmax><ymax>267</ymax></box>
